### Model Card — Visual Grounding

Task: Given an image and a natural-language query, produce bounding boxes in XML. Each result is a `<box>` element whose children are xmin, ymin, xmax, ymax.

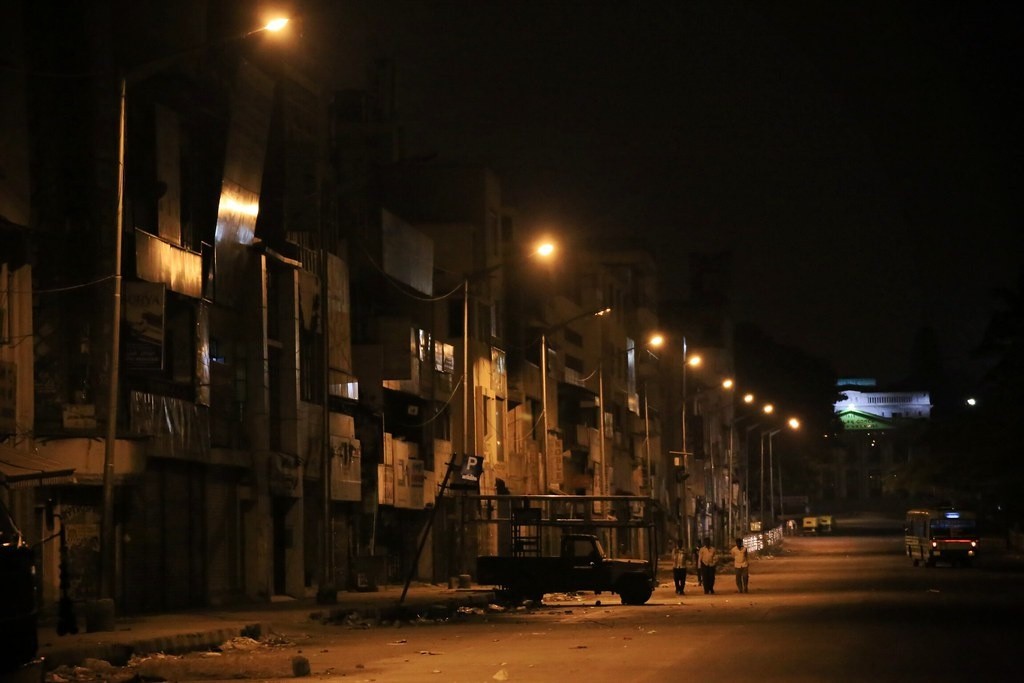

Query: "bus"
<box><xmin>902</xmin><ymin>509</ymin><xmax>981</xmax><ymax>569</ymax></box>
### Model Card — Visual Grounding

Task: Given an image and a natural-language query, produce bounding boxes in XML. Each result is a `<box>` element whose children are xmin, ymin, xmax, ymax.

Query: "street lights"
<box><xmin>460</xmin><ymin>237</ymin><xmax>564</xmax><ymax>577</ymax></box>
<box><xmin>94</xmin><ymin>13</ymin><xmax>292</xmax><ymax>602</ymax></box>
<box><xmin>759</xmin><ymin>418</ymin><xmax>799</xmax><ymax>536</ymax></box>
<box><xmin>708</xmin><ymin>393</ymin><xmax>755</xmax><ymax>549</ymax></box>
<box><xmin>727</xmin><ymin>405</ymin><xmax>776</xmax><ymax>548</ymax></box>
<box><xmin>598</xmin><ymin>334</ymin><xmax>665</xmax><ymax>559</ymax></box>
<box><xmin>644</xmin><ymin>356</ymin><xmax>703</xmax><ymax>560</ymax></box>
<box><xmin>680</xmin><ymin>378</ymin><xmax>734</xmax><ymax>553</ymax></box>
<box><xmin>541</xmin><ymin>303</ymin><xmax>612</xmax><ymax>558</ymax></box>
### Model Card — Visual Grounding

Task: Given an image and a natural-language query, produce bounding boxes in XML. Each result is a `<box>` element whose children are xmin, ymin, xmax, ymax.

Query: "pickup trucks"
<box><xmin>470</xmin><ymin>532</ymin><xmax>661</xmax><ymax>606</ymax></box>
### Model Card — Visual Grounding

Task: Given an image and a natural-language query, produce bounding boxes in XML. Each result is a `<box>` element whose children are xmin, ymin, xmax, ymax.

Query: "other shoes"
<box><xmin>698</xmin><ymin>582</ymin><xmax>701</xmax><ymax>586</ymax></box>
<box><xmin>711</xmin><ymin>590</ymin><xmax>714</xmax><ymax>594</ymax></box>
<box><xmin>704</xmin><ymin>590</ymin><xmax>709</xmax><ymax>594</ymax></box>
<box><xmin>676</xmin><ymin>589</ymin><xmax>685</xmax><ymax>595</ymax></box>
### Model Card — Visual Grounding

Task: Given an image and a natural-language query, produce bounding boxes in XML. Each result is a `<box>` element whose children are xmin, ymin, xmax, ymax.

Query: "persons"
<box><xmin>672</xmin><ymin>540</ymin><xmax>689</xmax><ymax>594</ymax></box>
<box><xmin>691</xmin><ymin>540</ymin><xmax>702</xmax><ymax>585</ymax></box>
<box><xmin>698</xmin><ymin>537</ymin><xmax>718</xmax><ymax>594</ymax></box>
<box><xmin>731</xmin><ymin>538</ymin><xmax>749</xmax><ymax>593</ymax></box>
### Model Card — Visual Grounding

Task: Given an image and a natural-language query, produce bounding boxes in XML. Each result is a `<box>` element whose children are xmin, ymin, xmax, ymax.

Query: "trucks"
<box><xmin>801</xmin><ymin>515</ymin><xmax>838</xmax><ymax>536</ymax></box>
